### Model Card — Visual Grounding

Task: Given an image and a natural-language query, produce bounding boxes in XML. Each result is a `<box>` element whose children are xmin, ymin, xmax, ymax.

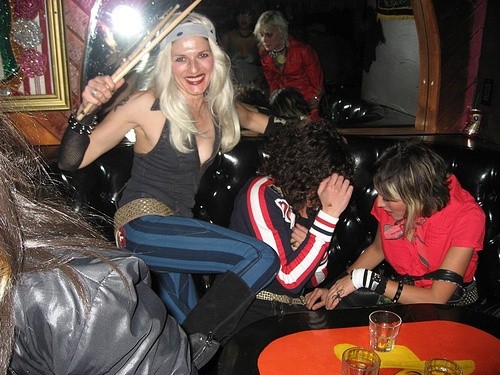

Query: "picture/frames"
<box><xmin>0</xmin><ymin>0</ymin><xmax>71</xmax><ymax>112</ymax></box>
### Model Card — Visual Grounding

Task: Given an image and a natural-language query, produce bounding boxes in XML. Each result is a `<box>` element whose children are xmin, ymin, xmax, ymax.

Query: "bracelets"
<box><xmin>68</xmin><ymin>111</ymin><xmax>98</xmax><ymax>135</ymax></box>
<box><xmin>393</xmin><ymin>280</ymin><xmax>404</xmax><ymax>303</ymax></box>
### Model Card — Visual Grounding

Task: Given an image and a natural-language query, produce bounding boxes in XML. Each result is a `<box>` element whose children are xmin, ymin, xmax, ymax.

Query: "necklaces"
<box><xmin>237</xmin><ymin>29</ymin><xmax>253</xmax><ymax>38</ymax></box>
<box><xmin>269</xmin><ymin>44</ymin><xmax>286</xmax><ymax>63</ymax></box>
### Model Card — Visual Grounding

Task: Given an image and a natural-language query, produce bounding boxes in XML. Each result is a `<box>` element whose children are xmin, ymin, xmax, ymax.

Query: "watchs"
<box><xmin>370</xmin><ymin>272</ymin><xmax>381</xmax><ymax>291</ymax></box>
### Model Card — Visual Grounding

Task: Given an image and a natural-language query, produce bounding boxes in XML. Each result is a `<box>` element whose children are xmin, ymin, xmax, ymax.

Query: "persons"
<box><xmin>58</xmin><ymin>13</ymin><xmax>290</xmax><ymax>375</ymax></box>
<box><xmin>0</xmin><ymin>112</ymin><xmax>196</xmax><ymax>375</ymax></box>
<box><xmin>225</xmin><ymin>122</ymin><xmax>359</xmax><ymax>340</ymax></box>
<box><xmin>88</xmin><ymin>4</ymin><xmax>325</xmax><ymax>121</ymax></box>
<box><xmin>305</xmin><ymin>140</ymin><xmax>486</xmax><ymax>310</ymax></box>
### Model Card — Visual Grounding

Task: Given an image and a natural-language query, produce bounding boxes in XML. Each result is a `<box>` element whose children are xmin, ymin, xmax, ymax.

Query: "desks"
<box><xmin>217</xmin><ymin>303</ymin><xmax>500</xmax><ymax>375</ymax></box>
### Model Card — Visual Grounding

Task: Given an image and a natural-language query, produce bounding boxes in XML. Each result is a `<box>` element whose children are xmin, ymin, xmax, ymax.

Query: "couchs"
<box><xmin>25</xmin><ymin>133</ymin><xmax>500</xmax><ymax>291</ymax></box>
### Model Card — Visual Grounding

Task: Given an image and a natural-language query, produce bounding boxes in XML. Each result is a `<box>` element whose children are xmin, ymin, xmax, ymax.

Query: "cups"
<box><xmin>424</xmin><ymin>358</ymin><xmax>463</xmax><ymax>375</ymax></box>
<box><xmin>368</xmin><ymin>310</ymin><xmax>402</xmax><ymax>352</ymax></box>
<box><xmin>341</xmin><ymin>347</ymin><xmax>381</xmax><ymax>375</ymax></box>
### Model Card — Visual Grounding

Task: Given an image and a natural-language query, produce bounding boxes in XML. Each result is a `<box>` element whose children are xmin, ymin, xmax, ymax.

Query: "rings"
<box><xmin>91</xmin><ymin>89</ymin><xmax>97</xmax><ymax>96</ymax></box>
<box><xmin>336</xmin><ymin>294</ymin><xmax>342</xmax><ymax>300</ymax></box>
